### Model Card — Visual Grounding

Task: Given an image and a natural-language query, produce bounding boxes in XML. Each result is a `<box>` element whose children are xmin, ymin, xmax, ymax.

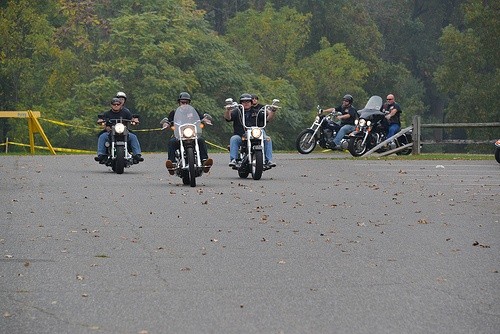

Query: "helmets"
<box><xmin>251</xmin><ymin>93</ymin><xmax>258</xmax><ymax>98</ymax></box>
<box><xmin>344</xmin><ymin>95</ymin><xmax>353</xmax><ymax>104</ymax></box>
<box><xmin>116</xmin><ymin>92</ymin><xmax>126</xmax><ymax>98</ymax></box>
<box><xmin>178</xmin><ymin>92</ymin><xmax>191</xmax><ymax>102</ymax></box>
<box><xmin>239</xmin><ymin>94</ymin><xmax>252</xmax><ymax>100</ymax></box>
<box><xmin>111</xmin><ymin>98</ymin><xmax>121</xmax><ymax>103</ymax></box>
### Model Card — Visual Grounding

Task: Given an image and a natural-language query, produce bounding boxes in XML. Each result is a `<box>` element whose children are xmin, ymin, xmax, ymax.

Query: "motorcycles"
<box><xmin>223</xmin><ymin>98</ymin><xmax>281</xmax><ymax>180</ymax></box>
<box><xmin>296</xmin><ymin>95</ymin><xmax>414</xmax><ymax>157</ymax></box>
<box><xmin>159</xmin><ymin>104</ymin><xmax>213</xmax><ymax>186</ymax></box>
<box><xmin>97</xmin><ymin>114</ymin><xmax>140</xmax><ymax>174</ymax></box>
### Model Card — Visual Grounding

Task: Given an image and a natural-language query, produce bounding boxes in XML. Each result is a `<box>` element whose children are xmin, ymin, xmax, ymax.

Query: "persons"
<box><xmin>95</xmin><ymin>92</ymin><xmax>143</xmax><ymax>161</ymax></box>
<box><xmin>323</xmin><ymin>95</ymin><xmax>359</xmax><ymax>151</ymax></box>
<box><xmin>225</xmin><ymin>94</ymin><xmax>277</xmax><ymax>167</ymax></box>
<box><xmin>163</xmin><ymin>92</ymin><xmax>213</xmax><ymax>175</ymax></box>
<box><xmin>380</xmin><ymin>94</ymin><xmax>402</xmax><ymax>147</ymax></box>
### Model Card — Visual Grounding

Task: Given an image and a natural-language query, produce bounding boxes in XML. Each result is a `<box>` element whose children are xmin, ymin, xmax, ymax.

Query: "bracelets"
<box><xmin>271</xmin><ymin>109</ymin><xmax>276</xmax><ymax>112</ymax></box>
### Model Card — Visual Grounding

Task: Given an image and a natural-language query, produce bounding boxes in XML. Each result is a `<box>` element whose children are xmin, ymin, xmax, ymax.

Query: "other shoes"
<box><xmin>165</xmin><ymin>160</ymin><xmax>174</xmax><ymax>176</ymax></box>
<box><xmin>265</xmin><ymin>160</ymin><xmax>276</xmax><ymax>167</ymax></box>
<box><xmin>202</xmin><ymin>158</ymin><xmax>213</xmax><ymax>173</ymax></box>
<box><xmin>94</xmin><ymin>155</ymin><xmax>108</xmax><ymax>161</ymax></box>
<box><xmin>325</xmin><ymin>141</ymin><xmax>336</xmax><ymax>147</ymax></box>
<box><xmin>136</xmin><ymin>157</ymin><xmax>144</xmax><ymax>164</ymax></box>
<box><xmin>229</xmin><ymin>159</ymin><xmax>236</xmax><ymax>167</ymax></box>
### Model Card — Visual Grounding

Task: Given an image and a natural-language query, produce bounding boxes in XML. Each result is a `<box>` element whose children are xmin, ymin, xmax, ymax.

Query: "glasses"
<box><xmin>112</xmin><ymin>103</ymin><xmax>121</xmax><ymax>106</ymax></box>
<box><xmin>252</xmin><ymin>97</ymin><xmax>258</xmax><ymax>100</ymax></box>
<box><xmin>180</xmin><ymin>101</ymin><xmax>189</xmax><ymax>104</ymax></box>
<box><xmin>241</xmin><ymin>100</ymin><xmax>251</xmax><ymax>103</ymax></box>
<box><xmin>343</xmin><ymin>99</ymin><xmax>349</xmax><ymax>102</ymax></box>
<box><xmin>386</xmin><ymin>99</ymin><xmax>393</xmax><ymax>100</ymax></box>
